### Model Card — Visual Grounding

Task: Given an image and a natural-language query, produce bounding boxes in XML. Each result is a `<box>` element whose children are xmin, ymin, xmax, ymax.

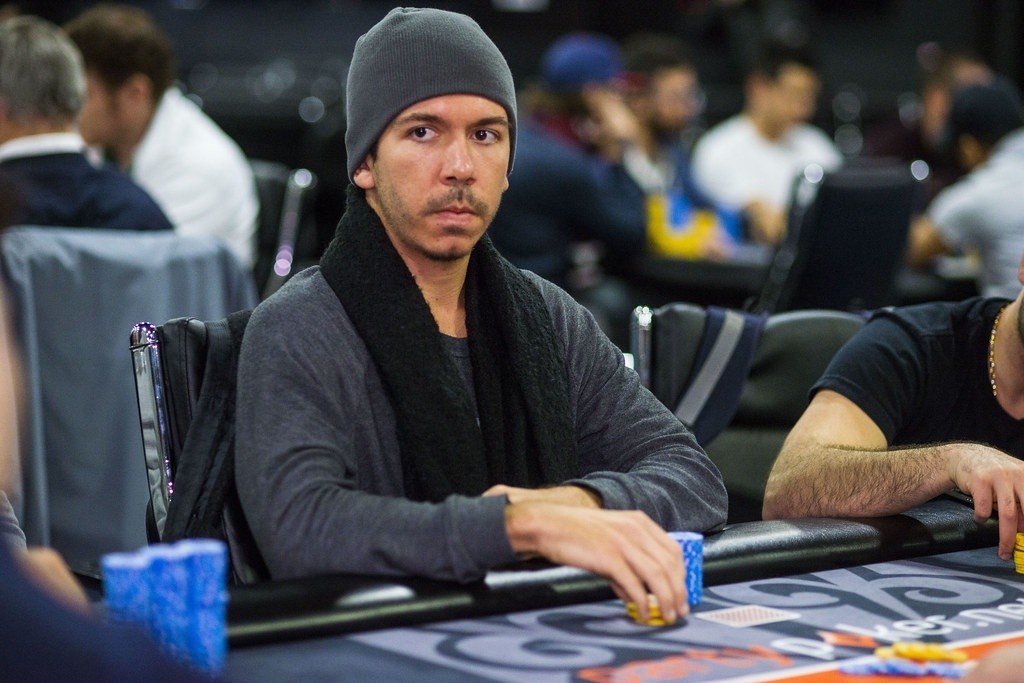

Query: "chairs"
<box><xmin>631</xmin><ymin>302</ymin><xmax>874</xmax><ymax>524</ymax></box>
<box><xmin>129</xmin><ymin>309</ymin><xmax>268</xmax><ymax>586</ymax></box>
<box><xmin>0</xmin><ymin>226</ymin><xmax>257</xmax><ymax>580</ymax></box>
<box><xmin>751</xmin><ymin>157</ymin><xmax>915</xmax><ymax>313</ymax></box>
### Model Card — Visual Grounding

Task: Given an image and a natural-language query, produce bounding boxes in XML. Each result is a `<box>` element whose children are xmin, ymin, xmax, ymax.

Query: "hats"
<box><xmin>539</xmin><ymin>33</ymin><xmax>627</xmax><ymax>82</ymax></box>
<box><xmin>342</xmin><ymin>7</ymin><xmax>520</xmax><ymax>187</ymax></box>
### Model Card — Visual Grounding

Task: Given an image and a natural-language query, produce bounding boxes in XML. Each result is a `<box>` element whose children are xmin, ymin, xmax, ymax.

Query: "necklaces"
<box><xmin>988</xmin><ymin>304</ymin><xmax>1008</xmax><ymax>398</ymax></box>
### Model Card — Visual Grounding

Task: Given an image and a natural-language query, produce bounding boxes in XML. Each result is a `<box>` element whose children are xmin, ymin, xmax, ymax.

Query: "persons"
<box><xmin>0</xmin><ymin>319</ymin><xmax>208</xmax><ymax>683</ymax></box>
<box><xmin>233</xmin><ymin>5</ymin><xmax>730</xmax><ymax>625</ymax></box>
<box><xmin>762</xmin><ymin>251</ymin><xmax>1024</xmax><ymax>561</ymax></box>
<box><xmin>625</xmin><ymin>68</ymin><xmax>739</xmax><ymax>258</ymax></box>
<box><xmin>506</xmin><ymin>44</ymin><xmax>649</xmax><ymax>288</ymax></box>
<box><xmin>905</xmin><ymin>81</ymin><xmax>1024</xmax><ymax>299</ymax></box>
<box><xmin>690</xmin><ymin>53</ymin><xmax>847</xmax><ymax>240</ymax></box>
<box><xmin>67</xmin><ymin>12</ymin><xmax>260</xmax><ymax>266</ymax></box>
<box><xmin>0</xmin><ymin>19</ymin><xmax>176</xmax><ymax>234</ymax></box>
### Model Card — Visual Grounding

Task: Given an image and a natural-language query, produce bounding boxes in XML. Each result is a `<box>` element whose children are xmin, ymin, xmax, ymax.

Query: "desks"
<box><xmin>226</xmin><ymin>495</ymin><xmax>1024</xmax><ymax>683</ymax></box>
